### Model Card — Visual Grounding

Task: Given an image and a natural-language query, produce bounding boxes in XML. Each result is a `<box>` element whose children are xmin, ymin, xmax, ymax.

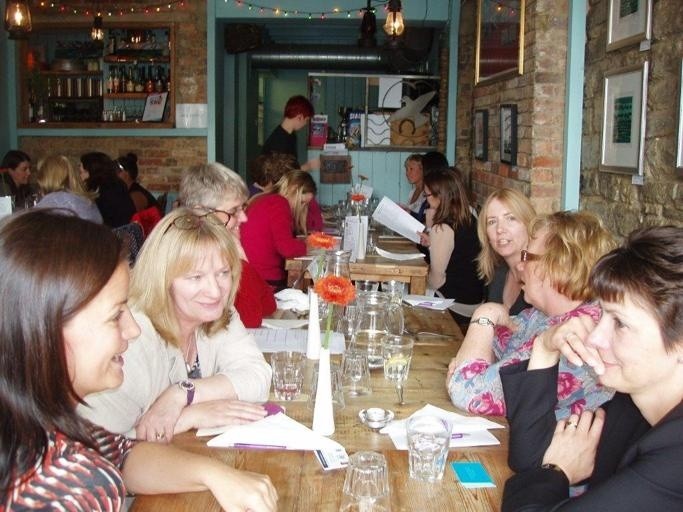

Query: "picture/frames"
<box><xmin>473</xmin><ymin>1</ymin><xmax>526</xmax><ymax>86</ymax></box>
<box><xmin>598</xmin><ymin>0</ymin><xmax>654</xmax><ymax>177</ymax></box>
<box><xmin>473</xmin><ymin>102</ymin><xmax>518</xmax><ymax>166</ymax></box>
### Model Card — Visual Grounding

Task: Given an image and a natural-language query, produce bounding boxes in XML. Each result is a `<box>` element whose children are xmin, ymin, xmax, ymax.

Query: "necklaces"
<box><xmin>180</xmin><ymin>334</ymin><xmax>194</xmax><ymax>372</ymax></box>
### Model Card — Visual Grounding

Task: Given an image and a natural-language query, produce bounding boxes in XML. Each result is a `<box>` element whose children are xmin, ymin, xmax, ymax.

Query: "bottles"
<box><xmin>101</xmin><ymin>103</ymin><xmax>146</xmax><ymax>122</ymax></box>
<box><xmin>106</xmin><ymin>64</ymin><xmax>171</xmax><ymax>95</ymax></box>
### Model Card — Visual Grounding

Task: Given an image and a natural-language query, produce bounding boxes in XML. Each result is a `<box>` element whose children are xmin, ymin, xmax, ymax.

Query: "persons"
<box><xmin>478</xmin><ymin>187</ymin><xmax>540</xmax><ymax>317</ymax></box>
<box><xmin>235</xmin><ymin>154</ymin><xmax>324</xmax><ymax>328</ymax></box>
<box><xmin>399</xmin><ymin>152</ymin><xmax>487</xmax><ymax>321</ymax></box>
<box><xmin>180</xmin><ymin>162</ymin><xmax>248</xmax><ymax>237</ymax></box>
<box><xmin>498</xmin><ymin>223</ymin><xmax>683</xmax><ymax>511</ymax></box>
<box><xmin>446</xmin><ymin>212</ymin><xmax>615</xmax><ymax>496</ymax></box>
<box><xmin>1</xmin><ymin>151</ymin><xmax>158</xmax><ymax>228</ymax></box>
<box><xmin>266</xmin><ymin>95</ymin><xmax>322</xmax><ymax>172</ymax></box>
<box><xmin>77</xmin><ymin>206</ymin><xmax>273</xmax><ymax>443</ymax></box>
<box><xmin>0</xmin><ymin>206</ymin><xmax>278</xmax><ymax>511</ymax></box>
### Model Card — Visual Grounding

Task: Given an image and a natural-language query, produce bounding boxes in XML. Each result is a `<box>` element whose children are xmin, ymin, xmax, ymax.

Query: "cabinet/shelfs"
<box><xmin>39</xmin><ymin>70</ymin><xmax>103</xmax><ymax>102</ymax></box>
<box><xmin>102</xmin><ymin>54</ymin><xmax>171</xmax><ymax>100</ymax></box>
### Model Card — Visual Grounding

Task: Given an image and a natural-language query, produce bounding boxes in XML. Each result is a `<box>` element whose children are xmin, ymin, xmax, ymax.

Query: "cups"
<box><xmin>404</xmin><ymin>413</ymin><xmax>455</xmax><ymax>484</ymax></box>
<box><xmin>376</xmin><ymin>334</ymin><xmax>417</xmax><ymax>380</ymax></box>
<box><xmin>338</xmin><ymin>350</ymin><xmax>375</xmax><ymax>398</ymax></box>
<box><xmin>346</xmin><ymin>290</ymin><xmax>405</xmax><ymax>371</ymax></box>
<box><xmin>268</xmin><ymin>349</ymin><xmax>307</xmax><ymax>399</ymax></box>
<box><xmin>338</xmin><ymin>196</ymin><xmax>383</xmax><ymax>253</ymax></box>
<box><xmin>321</xmin><ymin>246</ymin><xmax>354</xmax><ymax>285</ymax></box>
<box><xmin>340</xmin><ymin>449</ymin><xmax>391</xmax><ymax>512</ymax></box>
<box><xmin>380</xmin><ymin>280</ymin><xmax>406</xmax><ymax>292</ymax></box>
<box><xmin>354</xmin><ymin>278</ymin><xmax>379</xmax><ymax>291</ymax></box>
<box><xmin>306</xmin><ymin>361</ymin><xmax>346</xmax><ymax>417</ymax></box>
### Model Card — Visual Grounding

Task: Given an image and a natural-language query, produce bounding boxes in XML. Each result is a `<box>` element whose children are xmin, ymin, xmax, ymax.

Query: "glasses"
<box><xmin>422</xmin><ymin>191</ymin><xmax>432</xmax><ymax>197</ymax></box>
<box><xmin>163</xmin><ymin>210</ymin><xmax>231</xmax><ymax>233</ymax></box>
<box><xmin>227</xmin><ymin>203</ymin><xmax>249</xmax><ymax>215</ymax></box>
<box><xmin>520</xmin><ymin>251</ymin><xmax>540</xmax><ymax>263</ymax></box>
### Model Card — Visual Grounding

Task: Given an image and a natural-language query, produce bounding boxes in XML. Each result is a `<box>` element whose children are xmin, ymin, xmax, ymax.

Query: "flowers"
<box><xmin>314</xmin><ymin>274</ymin><xmax>357</xmax><ymax>349</ymax></box>
<box><xmin>352</xmin><ymin>172</ymin><xmax>369</xmax><ymax>223</ymax></box>
<box><xmin>306</xmin><ymin>230</ymin><xmax>337</xmax><ymax>277</ymax></box>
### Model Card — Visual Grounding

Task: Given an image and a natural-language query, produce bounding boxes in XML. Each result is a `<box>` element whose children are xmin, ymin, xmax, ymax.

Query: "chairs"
<box><xmin>109</xmin><ymin>190</ymin><xmax>170</xmax><ymax>266</ymax></box>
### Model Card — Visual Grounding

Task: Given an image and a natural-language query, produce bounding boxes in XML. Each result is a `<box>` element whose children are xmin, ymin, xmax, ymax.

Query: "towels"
<box><xmin>402</xmin><ymin>297</ymin><xmax>457</xmax><ymax>311</ymax></box>
<box><xmin>374</xmin><ymin>245</ymin><xmax>426</xmax><ymax>261</ymax></box>
<box><xmin>260</xmin><ymin>287</ymin><xmax>321</xmax><ymax>330</ymax></box>
<box><xmin>205</xmin><ymin>401</ymin><xmax>505</xmax><ymax>451</ymax></box>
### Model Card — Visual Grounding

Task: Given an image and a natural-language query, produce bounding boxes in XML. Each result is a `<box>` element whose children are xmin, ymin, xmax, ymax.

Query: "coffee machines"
<box><xmin>335</xmin><ymin>104</ymin><xmax>353</xmax><ymax>140</ymax></box>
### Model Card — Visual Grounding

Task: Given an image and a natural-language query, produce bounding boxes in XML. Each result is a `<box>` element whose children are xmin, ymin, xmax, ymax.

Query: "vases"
<box><xmin>306</xmin><ymin>288</ymin><xmax>322</xmax><ymax>360</ymax></box>
<box><xmin>355</xmin><ymin>223</ymin><xmax>366</xmax><ymax>259</ymax></box>
<box><xmin>311</xmin><ymin>349</ymin><xmax>336</xmax><ymax>438</ymax></box>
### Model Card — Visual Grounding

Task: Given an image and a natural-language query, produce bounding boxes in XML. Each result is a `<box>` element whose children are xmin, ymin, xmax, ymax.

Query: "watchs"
<box><xmin>470</xmin><ymin>317</ymin><xmax>496</xmax><ymax>330</ymax></box>
<box><xmin>176</xmin><ymin>379</ymin><xmax>196</xmax><ymax>405</ymax></box>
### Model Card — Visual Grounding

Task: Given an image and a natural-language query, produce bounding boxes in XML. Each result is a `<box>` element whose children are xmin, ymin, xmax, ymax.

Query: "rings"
<box><xmin>157</xmin><ymin>434</ymin><xmax>164</xmax><ymax>438</ymax></box>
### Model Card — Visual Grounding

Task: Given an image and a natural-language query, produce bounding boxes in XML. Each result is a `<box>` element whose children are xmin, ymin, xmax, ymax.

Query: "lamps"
<box><xmin>3</xmin><ymin>0</ymin><xmax>33</xmax><ymax>41</ymax></box>
<box><xmin>90</xmin><ymin>17</ymin><xmax>104</xmax><ymax>43</ymax></box>
<box><xmin>360</xmin><ymin>0</ymin><xmax>377</xmax><ymax>34</ymax></box>
<box><xmin>383</xmin><ymin>0</ymin><xmax>405</xmax><ymax>37</ymax></box>
